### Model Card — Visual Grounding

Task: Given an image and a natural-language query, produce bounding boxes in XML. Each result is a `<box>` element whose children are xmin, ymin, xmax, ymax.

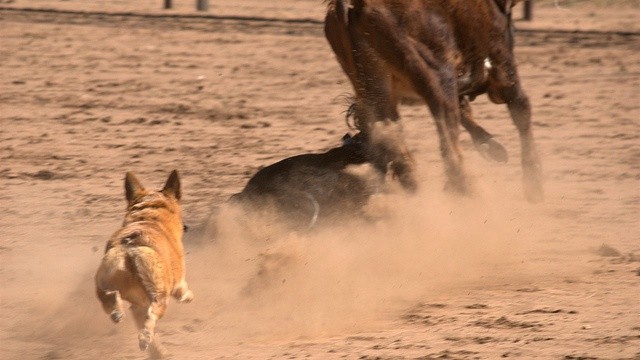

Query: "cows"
<box><xmin>321</xmin><ymin>0</ymin><xmax>546</xmax><ymax>205</ymax></box>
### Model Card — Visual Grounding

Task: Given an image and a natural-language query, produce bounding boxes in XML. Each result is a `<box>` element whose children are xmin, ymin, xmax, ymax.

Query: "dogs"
<box><xmin>94</xmin><ymin>169</ymin><xmax>195</xmax><ymax>360</ymax></box>
<box><xmin>234</xmin><ymin>132</ymin><xmax>395</xmax><ymax>241</ymax></box>
